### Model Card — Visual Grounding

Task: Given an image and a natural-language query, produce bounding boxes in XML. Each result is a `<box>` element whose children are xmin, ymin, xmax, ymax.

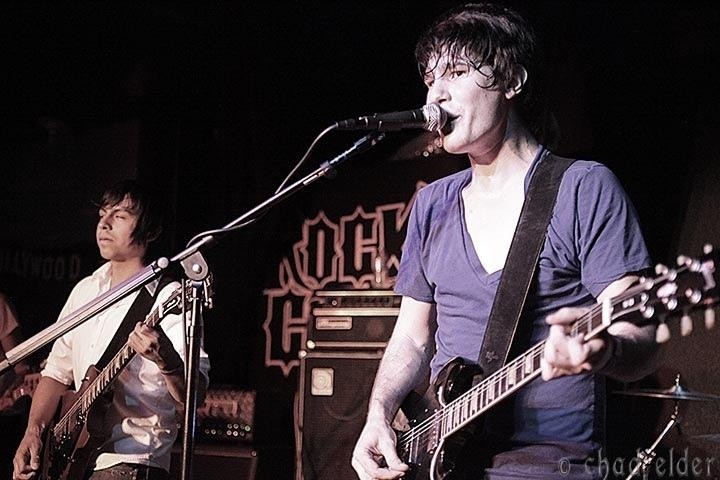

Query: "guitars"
<box><xmin>33</xmin><ymin>268</ymin><xmax>217</xmax><ymax>477</ymax></box>
<box><xmin>374</xmin><ymin>241</ymin><xmax>718</xmax><ymax>480</ymax></box>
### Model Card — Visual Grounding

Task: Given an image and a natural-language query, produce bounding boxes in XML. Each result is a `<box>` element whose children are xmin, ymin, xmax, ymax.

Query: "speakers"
<box><xmin>295</xmin><ymin>351</ymin><xmax>432</xmax><ymax>480</ymax></box>
<box><xmin>148</xmin><ymin>448</ymin><xmax>259</xmax><ymax>480</ymax></box>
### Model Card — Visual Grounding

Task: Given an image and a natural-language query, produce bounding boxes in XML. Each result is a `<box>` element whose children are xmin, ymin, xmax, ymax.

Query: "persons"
<box><xmin>350</xmin><ymin>4</ymin><xmax>665</xmax><ymax>480</ymax></box>
<box><xmin>0</xmin><ymin>173</ymin><xmax>211</xmax><ymax>480</ymax></box>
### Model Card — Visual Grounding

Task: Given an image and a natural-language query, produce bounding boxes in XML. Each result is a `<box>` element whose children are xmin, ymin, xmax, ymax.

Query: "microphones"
<box><xmin>336</xmin><ymin>101</ymin><xmax>448</xmax><ymax>133</ymax></box>
